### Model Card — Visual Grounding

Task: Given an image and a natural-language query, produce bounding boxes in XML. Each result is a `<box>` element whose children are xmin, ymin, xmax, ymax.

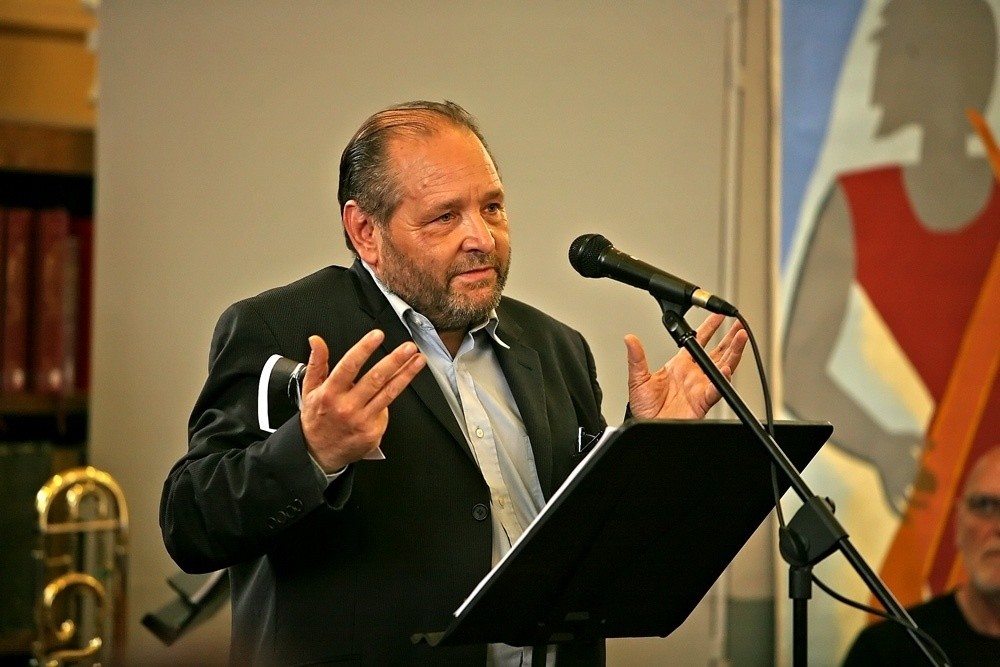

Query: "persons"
<box><xmin>839</xmin><ymin>445</ymin><xmax>1000</xmax><ymax>667</ymax></box>
<box><xmin>157</xmin><ymin>100</ymin><xmax>749</xmax><ymax>667</ymax></box>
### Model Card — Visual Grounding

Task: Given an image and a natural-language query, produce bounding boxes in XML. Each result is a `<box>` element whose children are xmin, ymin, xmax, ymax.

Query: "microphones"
<box><xmin>569</xmin><ymin>233</ymin><xmax>738</xmax><ymax>317</ymax></box>
